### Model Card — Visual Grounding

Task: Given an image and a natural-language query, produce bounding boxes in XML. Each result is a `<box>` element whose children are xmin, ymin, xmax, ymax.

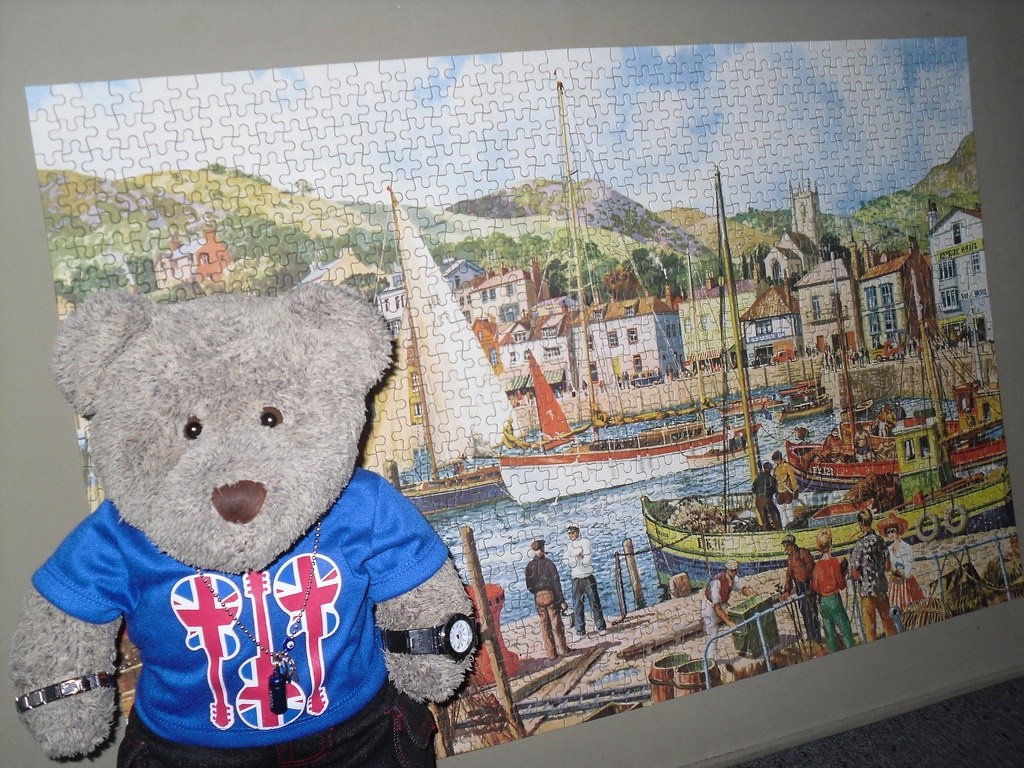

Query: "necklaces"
<box><xmin>196</xmin><ymin>522</ymin><xmax>321</xmax><ymax>715</ymax></box>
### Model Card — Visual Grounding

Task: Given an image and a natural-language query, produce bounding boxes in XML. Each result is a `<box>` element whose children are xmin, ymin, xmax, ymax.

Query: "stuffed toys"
<box><xmin>0</xmin><ymin>282</ymin><xmax>476</xmax><ymax>768</ymax></box>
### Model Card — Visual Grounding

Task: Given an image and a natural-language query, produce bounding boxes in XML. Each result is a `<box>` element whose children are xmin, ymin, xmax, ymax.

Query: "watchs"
<box><xmin>383</xmin><ymin>613</ymin><xmax>476</xmax><ymax>660</ymax></box>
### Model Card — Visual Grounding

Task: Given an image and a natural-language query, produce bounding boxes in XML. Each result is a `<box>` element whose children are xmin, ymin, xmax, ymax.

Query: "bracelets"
<box><xmin>14</xmin><ymin>671</ymin><xmax>113</xmax><ymax>714</ymax></box>
